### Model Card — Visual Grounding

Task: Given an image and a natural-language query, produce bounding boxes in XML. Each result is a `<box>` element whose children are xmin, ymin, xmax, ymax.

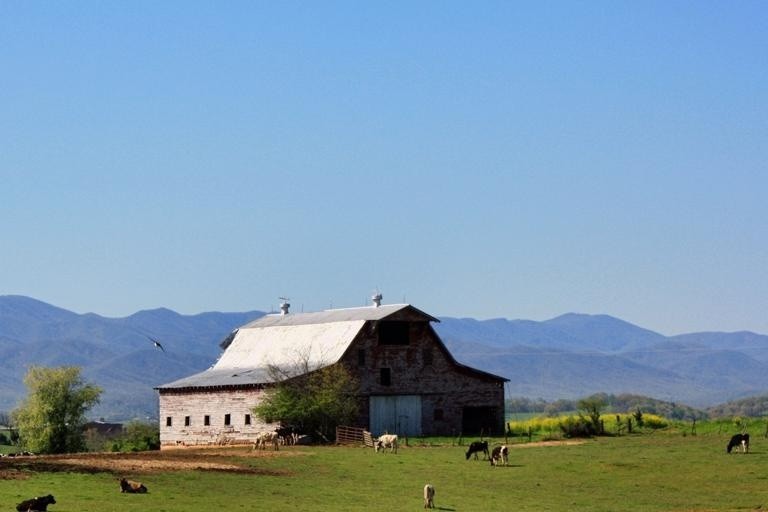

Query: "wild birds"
<box><xmin>145</xmin><ymin>335</ymin><xmax>165</xmax><ymax>352</ymax></box>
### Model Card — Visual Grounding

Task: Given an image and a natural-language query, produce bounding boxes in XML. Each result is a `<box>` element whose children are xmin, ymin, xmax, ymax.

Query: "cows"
<box><xmin>15</xmin><ymin>494</ymin><xmax>56</xmax><ymax>512</ymax></box>
<box><xmin>119</xmin><ymin>478</ymin><xmax>147</xmax><ymax>493</ymax></box>
<box><xmin>464</xmin><ymin>441</ymin><xmax>490</xmax><ymax>461</ymax></box>
<box><xmin>726</xmin><ymin>433</ymin><xmax>749</xmax><ymax>454</ymax></box>
<box><xmin>273</xmin><ymin>425</ymin><xmax>298</xmax><ymax>447</ymax></box>
<box><xmin>423</xmin><ymin>484</ymin><xmax>435</xmax><ymax>509</ymax></box>
<box><xmin>374</xmin><ymin>434</ymin><xmax>399</xmax><ymax>454</ymax></box>
<box><xmin>489</xmin><ymin>446</ymin><xmax>509</xmax><ymax>467</ymax></box>
<box><xmin>253</xmin><ymin>431</ymin><xmax>279</xmax><ymax>451</ymax></box>
